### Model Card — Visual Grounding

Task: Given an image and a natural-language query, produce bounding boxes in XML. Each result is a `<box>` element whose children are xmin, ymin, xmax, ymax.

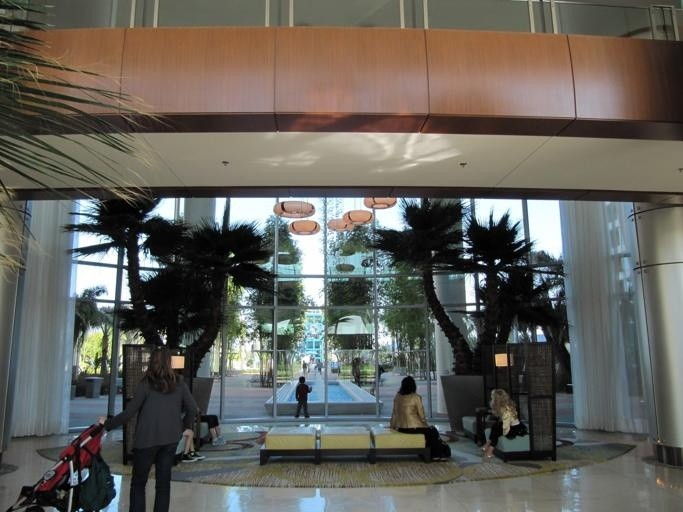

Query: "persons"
<box><xmin>181</xmin><ymin>428</ymin><xmax>205</xmax><ymax>462</ymax></box>
<box><xmin>200</xmin><ymin>414</ymin><xmax>227</xmax><ymax>446</ymax></box>
<box><xmin>479</xmin><ymin>388</ymin><xmax>528</xmax><ymax>459</ymax></box>
<box><xmin>98</xmin><ymin>345</ymin><xmax>195</xmax><ymax>511</ymax></box>
<box><xmin>295</xmin><ymin>376</ymin><xmax>312</xmax><ymax>418</ymax></box>
<box><xmin>315</xmin><ymin>358</ymin><xmax>323</xmax><ymax>374</ymax></box>
<box><xmin>302</xmin><ymin>360</ymin><xmax>313</xmax><ymax>376</ymax></box>
<box><xmin>351</xmin><ymin>357</ymin><xmax>361</xmax><ymax>387</ymax></box>
<box><xmin>391</xmin><ymin>376</ymin><xmax>451</xmax><ymax>462</ymax></box>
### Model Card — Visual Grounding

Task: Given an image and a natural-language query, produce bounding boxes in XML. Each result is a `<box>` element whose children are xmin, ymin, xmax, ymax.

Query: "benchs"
<box><xmin>260</xmin><ymin>423</ymin><xmax>433</xmax><ymax>466</ymax></box>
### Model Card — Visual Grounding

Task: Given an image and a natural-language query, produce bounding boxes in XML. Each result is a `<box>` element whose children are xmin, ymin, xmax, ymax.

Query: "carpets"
<box><xmin>30</xmin><ymin>423</ymin><xmax>640</xmax><ymax>491</ymax></box>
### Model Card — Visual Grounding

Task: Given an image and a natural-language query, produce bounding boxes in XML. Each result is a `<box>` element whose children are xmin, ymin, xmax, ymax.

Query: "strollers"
<box><xmin>8</xmin><ymin>416</ymin><xmax>116</xmax><ymax>512</ymax></box>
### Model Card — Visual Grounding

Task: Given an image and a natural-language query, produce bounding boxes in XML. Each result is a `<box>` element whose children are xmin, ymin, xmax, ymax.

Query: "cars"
<box><xmin>318</xmin><ymin>361</ymin><xmax>340</xmax><ymax>372</ymax></box>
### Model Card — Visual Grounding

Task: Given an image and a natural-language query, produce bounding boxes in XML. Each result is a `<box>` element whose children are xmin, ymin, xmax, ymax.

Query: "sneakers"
<box><xmin>212</xmin><ymin>439</ymin><xmax>224</xmax><ymax>446</ymax></box>
<box><xmin>190</xmin><ymin>451</ymin><xmax>205</xmax><ymax>460</ymax></box>
<box><xmin>181</xmin><ymin>453</ymin><xmax>197</xmax><ymax>463</ymax></box>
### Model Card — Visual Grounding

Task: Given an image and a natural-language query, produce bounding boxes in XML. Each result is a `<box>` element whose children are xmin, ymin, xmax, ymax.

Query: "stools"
<box><xmin>461</xmin><ymin>414</ymin><xmax>532</xmax><ymax>460</ymax></box>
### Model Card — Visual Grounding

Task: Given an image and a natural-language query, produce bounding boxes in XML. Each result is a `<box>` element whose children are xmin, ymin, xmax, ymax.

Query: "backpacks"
<box><xmin>77</xmin><ymin>443</ymin><xmax>116</xmax><ymax>509</ymax></box>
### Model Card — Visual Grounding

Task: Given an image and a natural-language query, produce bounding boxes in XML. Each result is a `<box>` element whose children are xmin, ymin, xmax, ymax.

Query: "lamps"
<box><xmin>273</xmin><ymin>196</ymin><xmax>399</xmax><ymax>236</ymax></box>
